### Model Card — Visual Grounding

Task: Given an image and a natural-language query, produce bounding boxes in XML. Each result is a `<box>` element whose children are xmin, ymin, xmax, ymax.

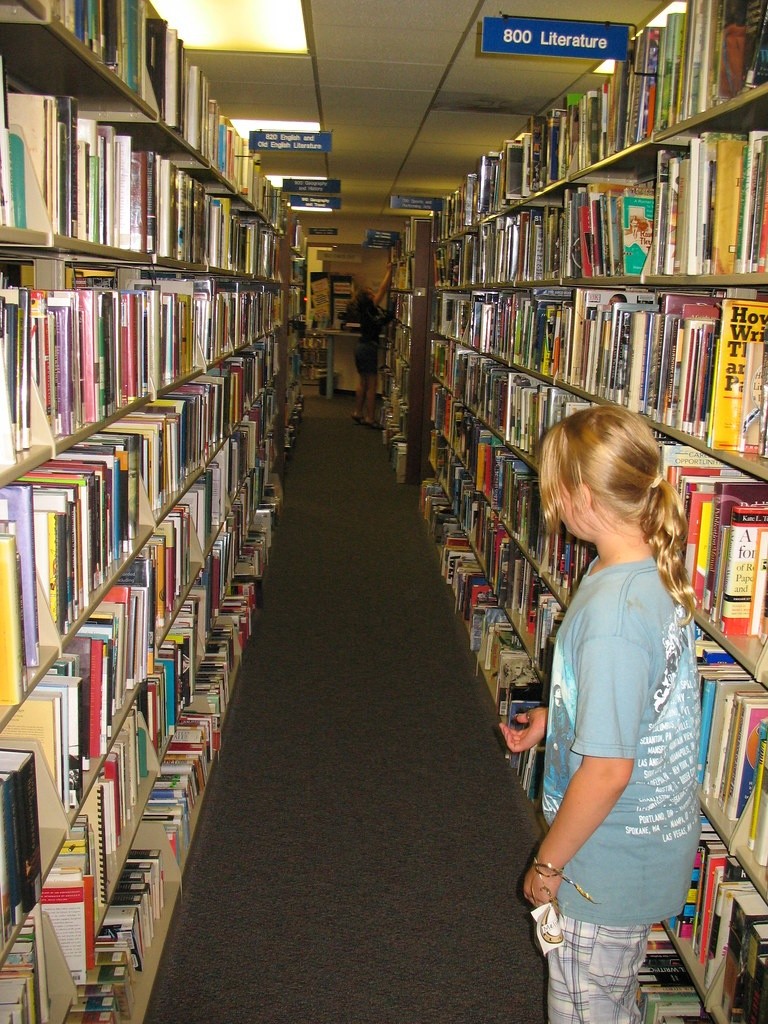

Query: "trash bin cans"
<box><xmin>318</xmin><ymin>370</ymin><xmax>336</xmax><ymax>395</ymax></box>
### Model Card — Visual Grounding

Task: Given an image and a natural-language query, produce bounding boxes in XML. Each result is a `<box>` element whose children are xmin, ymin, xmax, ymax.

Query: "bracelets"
<box><xmin>533</xmin><ymin>856</ymin><xmax>563</xmax><ymax>877</ymax></box>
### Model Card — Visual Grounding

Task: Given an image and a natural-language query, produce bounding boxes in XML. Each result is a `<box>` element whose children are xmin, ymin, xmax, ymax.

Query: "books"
<box><xmin>0</xmin><ymin>0</ymin><xmax>768</xmax><ymax>1024</ymax></box>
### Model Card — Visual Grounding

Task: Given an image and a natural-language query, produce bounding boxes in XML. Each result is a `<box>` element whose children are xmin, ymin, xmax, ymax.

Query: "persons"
<box><xmin>352</xmin><ymin>263</ymin><xmax>392</xmax><ymax>426</ymax></box>
<box><xmin>550</xmin><ymin>683</ymin><xmax>573</xmax><ymax>793</ymax></box>
<box><xmin>499</xmin><ymin>406</ymin><xmax>700</xmax><ymax>1024</ymax></box>
<box><xmin>607</xmin><ymin>294</ymin><xmax>627</xmax><ymax>304</ymax></box>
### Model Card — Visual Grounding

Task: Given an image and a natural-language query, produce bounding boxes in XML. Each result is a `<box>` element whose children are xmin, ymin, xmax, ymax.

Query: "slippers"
<box><xmin>351</xmin><ymin>414</ymin><xmax>384</xmax><ymax>430</ymax></box>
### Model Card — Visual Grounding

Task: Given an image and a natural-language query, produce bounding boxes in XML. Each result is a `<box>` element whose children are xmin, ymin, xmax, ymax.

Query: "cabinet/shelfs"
<box><xmin>366</xmin><ymin>0</ymin><xmax>768</xmax><ymax>1024</ymax></box>
<box><xmin>0</xmin><ymin>0</ymin><xmax>307</xmax><ymax>1024</ymax></box>
